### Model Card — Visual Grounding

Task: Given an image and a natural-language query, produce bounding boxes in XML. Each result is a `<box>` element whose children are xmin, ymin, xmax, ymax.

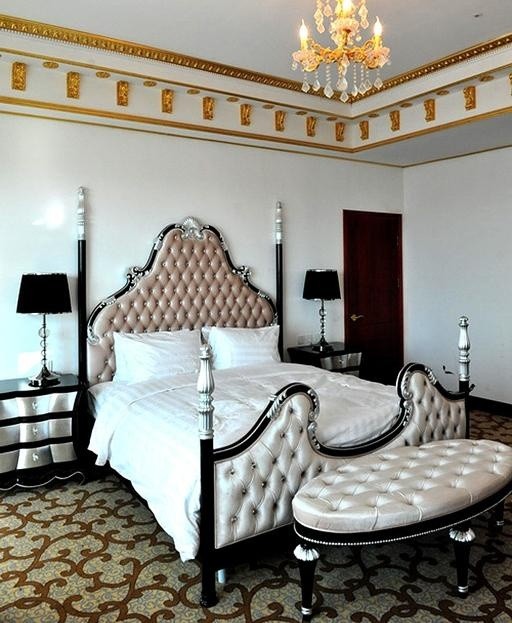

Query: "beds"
<box><xmin>83</xmin><ymin>217</ymin><xmax>474</xmax><ymax>610</ymax></box>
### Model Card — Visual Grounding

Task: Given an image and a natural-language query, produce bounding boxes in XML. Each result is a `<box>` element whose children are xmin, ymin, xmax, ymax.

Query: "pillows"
<box><xmin>111</xmin><ymin>324</ymin><xmax>290</xmax><ymax>381</ymax></box>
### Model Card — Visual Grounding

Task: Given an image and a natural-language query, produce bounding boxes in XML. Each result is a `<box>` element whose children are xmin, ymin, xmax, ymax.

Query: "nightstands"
<box><xmin>287</xmin><ymin>342</ymin><xmax>364</xmax><ymax>380</ymax></box>
<box><xmin>0</xmin><ymin>371</ymin><xmax>88</xmax><ymax>493</ymax></box>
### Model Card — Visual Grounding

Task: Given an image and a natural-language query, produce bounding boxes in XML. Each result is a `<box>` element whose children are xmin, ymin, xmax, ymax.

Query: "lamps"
<box><xmin>287</xmin><ymin>0</ymin><xmax>393</xmax><ymax>106</ymax></box>
<box><xmin>303</xmin><ymin>268</ymin><xmax>342</xmax><ymax>353</ymax></box>
<box><xmin>17</xmin><ymin>270</ymin><xmax>73</xmax><ymax>388</ymax></box>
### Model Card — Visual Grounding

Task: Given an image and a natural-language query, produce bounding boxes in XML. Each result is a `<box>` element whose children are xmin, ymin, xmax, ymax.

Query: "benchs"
<box><xmin>291</xmin><ymin>437</ymin><xmax>512</xmax><ymax>623</ymax></box>
